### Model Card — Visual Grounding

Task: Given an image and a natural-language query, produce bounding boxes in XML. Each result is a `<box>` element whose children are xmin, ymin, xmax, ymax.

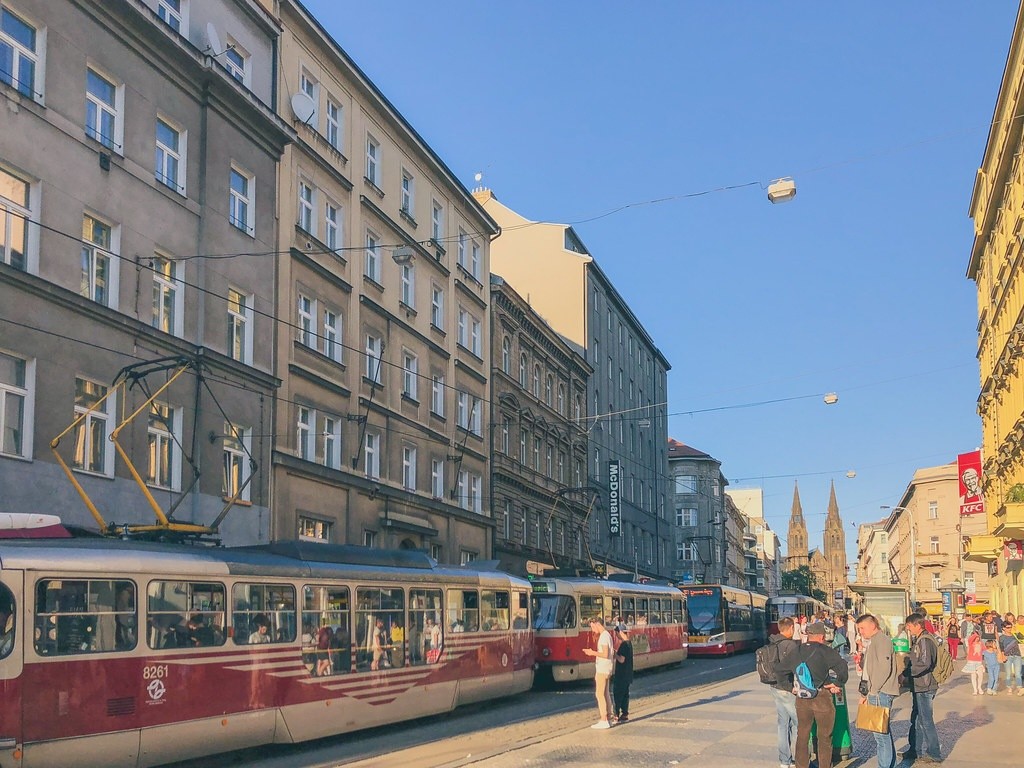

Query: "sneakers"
<box><xmin>781</xmin><ymin>764</ymin><xmax>790</xmax><ymax>768</ymax></box>
<box><xmin>611</xmin><ymin>716</ymin><xmax>621</xmax><ymax>724</ymax></box>
<box><xmin>987</xmin><ymin>688</ymin><xmax>992</xmax><ymax>695</ymax></box>
<box><xmin>896</xmin><ymin>750</ymin><xmax>924</xmax><ymax>760</ymax></box>
<box><xmin>912</xmin><ymin>754</ymin><xmax>941</xmax><ymax>765</ymax></box>
<box><xmin>1018</xmin><ymin>688</ymin><xmax>1024</xmax><ymax>696</ymax></box>
<box><xmin>619</xmin><ymin>714</ymin><xmax>629</xmax><ymax>722</ymax></box>
<box><xmin>591</xmin><ymin>720</ymin><xmax>614</xmax><ymax>729</ymax></box>
<box><xmin>972</xmin><ymin>690</ymin><xmax>977</xmax><ymax>696</ymax></box>
<box><xmin>991</xmin><ymin>689</ymin><xmax>997</xmax><ymax>696</ymax></box>
<box><xmin>978</xmin><ymin>689</ymin><xmax>985</xmax><ymax>695</ymax></box>
<box><xmin>1007</xmin><ymin>687</ymin><xmax>1013</xmax><ymax>696</ymax></box>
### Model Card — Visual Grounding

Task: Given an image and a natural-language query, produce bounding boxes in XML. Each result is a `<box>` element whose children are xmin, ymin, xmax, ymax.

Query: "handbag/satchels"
<box><xmin>855</xmin><ymin>692</ymin><xmax>890</xmax><ymax>735</ymax></box>
<box><xmin>858</xmin><ymin>680</ymin><xmax>869</xmax><ymax>696</ymax></box>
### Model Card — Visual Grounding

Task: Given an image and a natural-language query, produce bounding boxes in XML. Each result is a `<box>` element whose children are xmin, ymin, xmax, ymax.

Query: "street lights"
<box><xmin>880</xmin><ymin>506</ymin><xmax>917</xmax><ymax>615</ymax></box>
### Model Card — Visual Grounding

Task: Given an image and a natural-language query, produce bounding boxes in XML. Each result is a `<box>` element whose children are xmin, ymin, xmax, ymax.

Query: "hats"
<box><xmin>614</xmin><ymin>624</ymin><xmax>631</xmax><ymax>633</ymax></box>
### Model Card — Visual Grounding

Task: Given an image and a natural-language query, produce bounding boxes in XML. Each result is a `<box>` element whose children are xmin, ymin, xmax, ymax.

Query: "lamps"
<box><xmin>764</xmin><ymin>179</ymin><xmax>796</xmax><ymax>207</ymax></box>
<box><xmin>389</xmin><ymin>240</ymin><xmax>418</xmax><ymax>267</ymax></box>
<box><xmin>846</xmin><ymin>470</ymin><xmax>856</xmax><ymax>480</ymax></box>
<box><xmin>822</xmin><ymin>393</ymin><xmax>841</xmax><ymax>409</ymax></box>
<box><xmin>638</xmin><ymin>416</ymin><xmax>653</xmax><ymax>427</ymax></box>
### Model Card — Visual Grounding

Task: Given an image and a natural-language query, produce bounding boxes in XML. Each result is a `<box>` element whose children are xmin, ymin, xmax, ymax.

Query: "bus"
<box><xmin>0</xmin><ymin>513</ymin><xmax>536</xmax><ymax>768</ymax></box>
<box><xmin>765</xmin><ymin>589</ymin><xmax>835</xmax><ymax>638</ymax></box>
<box><xmin>677</xmin><ymin>584</ymin><xmax>768</xmax><ymax>657</ymax></box>
<box><xmin>528</xmin><ymin>578</ymin><xmax>689</xmax><ymax>683</ymax></box>
<box><xmin>847</xmin><ymin>584</ymin><xmax>912</xmax><ymax>653</ymax></box>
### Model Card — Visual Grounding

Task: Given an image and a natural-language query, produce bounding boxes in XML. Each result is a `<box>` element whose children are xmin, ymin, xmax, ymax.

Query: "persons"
<box><xmin>981</xmin><ymin>639</ymin><xmax>1001</xmax><ymax>696</ymax></box>
<box><xmin>610</xmin><ymin>624</ymin><xmax>634</xmax><ymax>726</ymax></box>
<box><xmin>582</xmin><ymin>616</ymin><xmax>616</xmax><ymax>731</ymax></box>
<box><xmin>914</xmin><ymin>606</ymin><xmax>935</xmax><ymax>635</ymax></box>
<box><xmin>855</xmin><ymin>614</ymin><xmax>900</xmax><ymax>768</ymax></box>
<box><xmin>766</xmin><ymin>618</ymin><xmax>799</xmax><ymax>768</ymax></box>
<box><xmin>775</xmin><ymin>621</ymin><xmax>849</xmax><ymax>768</ymax></box>
<box><xmin>580</xmin><ymin>611</ymin><xmax>679</xmax><ymax>626</ymax></box>
<box><xmin>790</xmin><ymin>608</ymin><xmax>857</xmax><ymax>656</ymax></box>
<box><xmin>998</xmin><ymin>623</ymin><xmax>1024</xmax><ymax>697</ymax></box>
<box><xmin>29</xmin><ymin>596</ymin><xmax>533</xmax><ymax>680</ymax></box>
<box><xmin>897</xmin><ymin>613</ymin><xmax>942</xmax><ymax>764</ymax></box>
<box><xmin>943</xmin><ymin>607</ymin><xmax>1024</xmax><ymax>674</ymax></box>
<box><xmin>961</xmin><ymin>633</ymin><xmax>986</xmax><ymax>695</ymax></box>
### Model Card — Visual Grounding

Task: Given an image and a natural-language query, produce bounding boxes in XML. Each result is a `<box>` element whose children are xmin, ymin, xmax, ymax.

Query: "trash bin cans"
<box><xmin>891</xmin><ymin>638</ymin><xmax>911</xmax><ymax>688</ymax></box>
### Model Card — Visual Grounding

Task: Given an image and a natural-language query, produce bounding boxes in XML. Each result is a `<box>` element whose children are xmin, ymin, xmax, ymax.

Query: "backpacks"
<box><xmin>792</xmin><ymin>644</ymin><xmax>829</xmax><ymax>700</ymax></box>
<box><xmin>755</xmin><ymin>638</ymin><xmax>790</xmax><ymax>684</ymax></box>
<box><xmin>915</xmin><ymin>634</ymin><xmax>955</xmax><ymax>684</ymax></box>
